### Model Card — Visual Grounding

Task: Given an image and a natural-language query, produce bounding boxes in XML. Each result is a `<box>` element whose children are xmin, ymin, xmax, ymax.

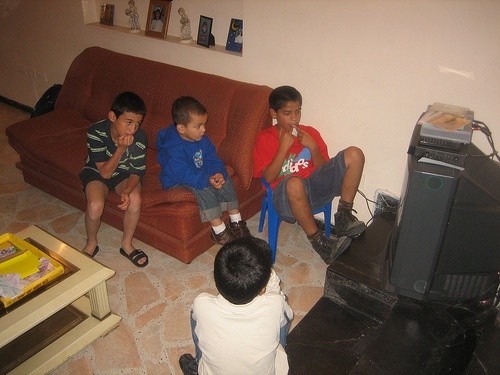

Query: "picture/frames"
<box><xmin>144</xmin><ymin>0</ymin><xmax>172</xmax><ymax>40</ymax></box>
<box><xmin>197</xmin><ymin>15</ymin><xmax>213</xmax><ymax>48</ymax></box>
<box><xmin>226</xmin><ymin>18</ymin><xmax>243</xmax><ymax>53</ymax></box>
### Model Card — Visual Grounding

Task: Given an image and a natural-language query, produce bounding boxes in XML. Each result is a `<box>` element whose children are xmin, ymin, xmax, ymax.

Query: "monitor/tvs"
<box><xmin>384</xmin><ymin>112</ymin><xmax>500</xmax><ymax>309</ymax></box>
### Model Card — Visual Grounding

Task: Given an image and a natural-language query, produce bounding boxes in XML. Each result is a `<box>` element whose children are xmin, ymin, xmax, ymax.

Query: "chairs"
<box><xmin>259</xmin><ymin>177</ymin><xmax>332</xmax><ymax>263</ymax></box>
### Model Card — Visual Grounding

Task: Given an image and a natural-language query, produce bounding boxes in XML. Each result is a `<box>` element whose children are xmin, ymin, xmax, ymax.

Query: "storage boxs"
<box><xmin>0</xmin><ymin>232</ymin><xmax>64</xmax><ymax>308</ymax></box>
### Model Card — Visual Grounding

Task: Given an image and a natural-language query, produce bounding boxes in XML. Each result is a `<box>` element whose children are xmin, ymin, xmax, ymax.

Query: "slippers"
<box><xmin>120</xmin><ymin>247</ymin><xmax>148</xmax><ymax>267</ymax></box>
<box><xmin>81</xmin><ymin>245</ymin><xmax>99</xmax><ymax>258</ymax></box>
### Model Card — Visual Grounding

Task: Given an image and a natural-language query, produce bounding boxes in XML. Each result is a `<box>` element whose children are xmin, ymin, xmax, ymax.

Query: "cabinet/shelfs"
<box><xmin>323</xmin><ymin>215</ymin><xmax>500</xmax><ymax>358</ymax></box>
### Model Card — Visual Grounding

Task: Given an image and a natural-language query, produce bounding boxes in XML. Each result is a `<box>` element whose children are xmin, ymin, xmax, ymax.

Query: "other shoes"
<box><xmin>180</xmin><ymin>353</ymin><xmax>198</xmax><ymax>375</ymax></box>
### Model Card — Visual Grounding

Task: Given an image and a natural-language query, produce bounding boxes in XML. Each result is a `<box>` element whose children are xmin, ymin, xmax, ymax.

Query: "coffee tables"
<box><xmin>0</xmin><ymin>224</ymin><xmax>122</xmax><ymax>375</ymax></box>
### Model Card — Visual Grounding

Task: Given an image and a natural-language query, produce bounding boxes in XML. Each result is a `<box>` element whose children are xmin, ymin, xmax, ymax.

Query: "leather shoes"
<box><xmin>312</xmin><ymin>234</ymin><xmax>352</xmax><ymax>265</ymax></box>
<box><xmin>334</xmin><ymin>206</ymin><xmax>365</xmax><ymax>236</ymax></box>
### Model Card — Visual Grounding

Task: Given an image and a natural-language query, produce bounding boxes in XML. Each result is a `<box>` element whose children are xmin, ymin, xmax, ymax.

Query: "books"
<box><xmin>418</xmin><ymin>102</ymin><xmax>472</xmax><ymax>133</ymax></box>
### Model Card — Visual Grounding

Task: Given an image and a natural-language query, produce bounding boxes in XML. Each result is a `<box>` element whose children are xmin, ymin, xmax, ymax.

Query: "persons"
<box><xmin>156</xmin><ymin>96</ymin><xmax>251</xmax><ymax>245</ymax></box>
<box><xmin>199</xmin><ymin>23</ymin><xmax>209</xmax><ymax>44</ymax></box>
<box><xmin>79</xmin><ymin>92</ymin><xmax>148</xmax><ymax>268</ymax></box>
<box><xmin>124</xmin><ymin>0</ymin><xmax>139</xmax><ymax>30</ymax></box>
<box><xmin>151</xmin><ymin>6</ymin><xmax>163</xmax><ymax>32</ymax></box>
<box><xmin>179</xmin><ymin>237</ymin><xmax>295</xmax><ymax>375</ymax></box>
<box><xmin>253</xmin><ymin>85</ymin><xmax>367</xmax><ymax>265</ymax></box>
<box><xmin>177</xmin><ymin>8</ymin><xmax>191</xmax><ymax>39</ymax></box>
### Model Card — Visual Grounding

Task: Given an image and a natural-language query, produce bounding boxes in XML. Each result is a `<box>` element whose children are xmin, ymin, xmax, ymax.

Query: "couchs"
<box><xmin>5</xmin><ymin>47</ymin><xmax>272</xmax><ymax>265</ymax></box>
<box><xmin>285</xmin><ymin>297</ymin><xmax>477</xmax><ymax>375</ymax></box>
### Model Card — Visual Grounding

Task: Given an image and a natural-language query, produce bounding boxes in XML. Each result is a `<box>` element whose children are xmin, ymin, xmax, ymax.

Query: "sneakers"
<box><xmin>225</xmin><ymin>218</ymin><xmax>250</xmax><ymax>239</ymax></box>
<box><xmin>210</xmin><ymin>225</ymin><xmax>235</xmax><ymax>245</ymax></box>
